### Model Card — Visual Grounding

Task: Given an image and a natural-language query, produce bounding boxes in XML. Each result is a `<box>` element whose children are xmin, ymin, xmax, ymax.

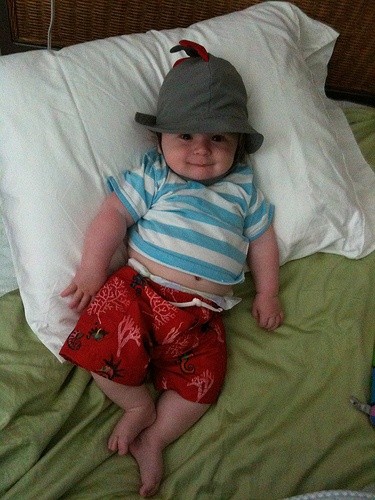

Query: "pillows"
<box><xmin>0</xmin><ymin>0</ymin><xmax>375</xmax><ymax>361</ymax></box>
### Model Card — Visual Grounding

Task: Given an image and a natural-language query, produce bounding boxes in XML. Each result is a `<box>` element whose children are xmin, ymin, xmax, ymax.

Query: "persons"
<box><xmin>59</xmin><ymin>38</ymin><xmax>282</xmax><ymax>497</ymax></box>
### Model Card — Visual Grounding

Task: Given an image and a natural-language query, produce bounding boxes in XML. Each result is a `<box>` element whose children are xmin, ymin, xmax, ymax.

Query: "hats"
<box><xmin>135</xmin><ymin>39</ymin><xmax>264</xmax><ymax>155</ymax></box>
